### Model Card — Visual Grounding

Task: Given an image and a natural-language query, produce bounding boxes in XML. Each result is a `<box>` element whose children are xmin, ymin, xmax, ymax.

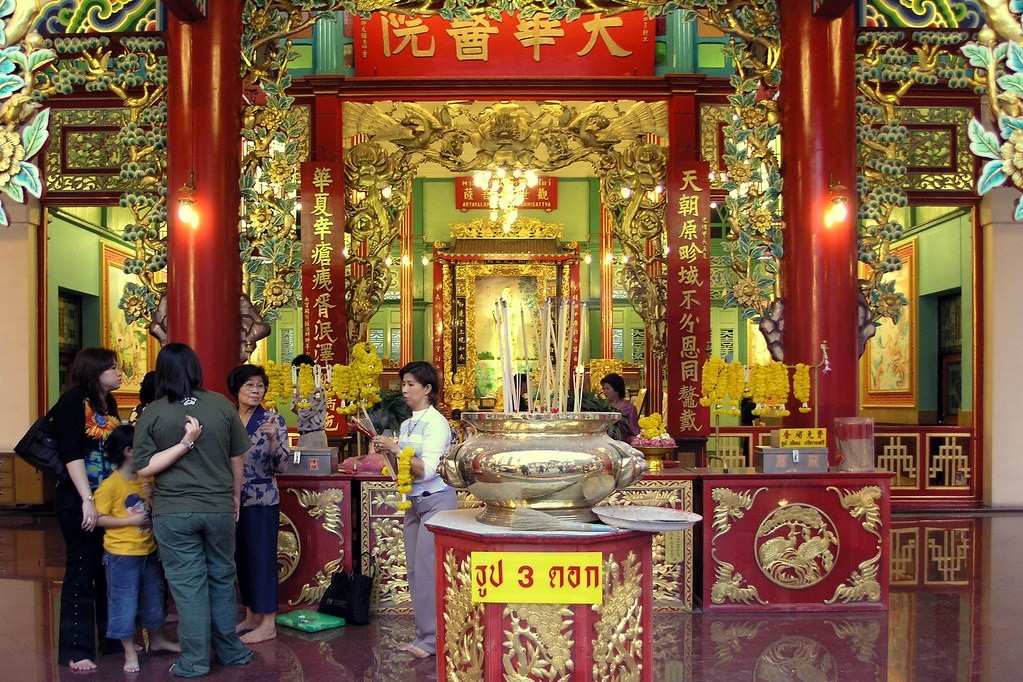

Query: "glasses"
<box><xmin>241</xmin><ymin>384</ymin><xmax>268</xmax><ymax>393</ymax></box>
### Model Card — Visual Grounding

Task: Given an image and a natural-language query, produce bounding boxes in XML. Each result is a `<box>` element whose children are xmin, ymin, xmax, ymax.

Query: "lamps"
<box><xmin>176</xmin><ymin>183</ymin><xmax>200</xmax><ymax>229</ymax></box>
<box><xmin>469</xmin><ymin>163</ymin><xmax>539</xmax><ymax>236</ymax></box>
<box><xmin>823</xmin><ymin>182</ymin><xmax>849</xmax><ymax>227</ymax></box>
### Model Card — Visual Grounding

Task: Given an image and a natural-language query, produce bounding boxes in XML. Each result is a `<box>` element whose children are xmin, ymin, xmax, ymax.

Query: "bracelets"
<box><xmin>396</xmin><ymin>447</ymin><xmax>403</xmax><ymax>459</ymax></box>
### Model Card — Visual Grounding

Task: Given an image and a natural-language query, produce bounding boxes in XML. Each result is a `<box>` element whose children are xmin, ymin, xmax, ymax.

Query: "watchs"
<box><xmin>180</xmin><ymin>439</ymin><xmax>195</xmax><ymax>449</ymax></box>
<box><xmin>82</xmin><ymin>495</ymin><xmax>95</xmax><ymax>501</ymax></box>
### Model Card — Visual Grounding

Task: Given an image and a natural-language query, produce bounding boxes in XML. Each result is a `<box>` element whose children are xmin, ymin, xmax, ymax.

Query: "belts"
<box><xmin>298</xmin><ymin>427</ymin><xmax>324</xmax><ymax>435</ymax></box>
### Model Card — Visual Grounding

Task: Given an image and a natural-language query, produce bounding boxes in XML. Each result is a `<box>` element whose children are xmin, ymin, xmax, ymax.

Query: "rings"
<box><xmin>375</xmin><ymin>444</ymin><xmax>378</xmax><ymax>447</ymax></box>
<box><xmin>87</xmin><ymin>517</ymin><xmax>91</xmax><ymax>524</ymax></box>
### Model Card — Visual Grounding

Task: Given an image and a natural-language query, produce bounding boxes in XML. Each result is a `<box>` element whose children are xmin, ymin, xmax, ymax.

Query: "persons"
<box><xmin>227</xmin><ymin>364</ymin><xmax>290</xmax><ymax>646</ymax></box>
<box><xmin>600</xmin><ymin>372</ymin><xmax>641</xmax><ymax>437</ymax></box>
<box><xmin>370</xmin><ymin>361</ymin><xmax>459</xmax><ymax>658</ymax></box>
<box><xmin>290</xmin><ymin>354</ymin><xmax>328</xmax><ymax>450</ymax></box>
<box><xmin>741</xmin><ymin>391</ymin><xmax>760</xmax><ymax>468</ymax></box>
<box><xmin>511</xmin><ymin>373</ymin><xmax>538</xmax><ymax>412</ymax></box>
<box><xmin>132</xmin><ymin>341</ymin><xmax>254</xmax><ymax>677</ymax></box>
<box><xmin>47</xmin><ymin>346</ymin><xmax>182</xmax><ymax>673</ymax></box>
<box><xmin>518</xmin><ymin>462</ymin><xmax>598</xmax><ymax>475</ymax></box>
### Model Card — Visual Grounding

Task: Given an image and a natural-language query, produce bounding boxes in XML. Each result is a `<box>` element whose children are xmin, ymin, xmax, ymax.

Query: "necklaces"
<box><xmin>407</xmin><ymin>406</ymin><xmax>429</xmax><ymax>437</ymax></box>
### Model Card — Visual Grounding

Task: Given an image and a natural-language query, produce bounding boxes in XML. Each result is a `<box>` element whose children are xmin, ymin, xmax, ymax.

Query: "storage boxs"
<box><xmin>754</xmin><ymin>444</ymin><xmax>830</xmax><ymax>474</ymax></box>
<box><xmin>285</xmin><ymin>445</ymin><xmax>340</xmax><ymax>474</ymax></box>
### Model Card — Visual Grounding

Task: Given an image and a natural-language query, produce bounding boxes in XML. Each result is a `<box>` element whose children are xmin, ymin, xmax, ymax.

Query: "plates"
<box><xmin>591</xmin><ymin>506</ymin><xmax>703</xmax><ymax>531</ymax></box>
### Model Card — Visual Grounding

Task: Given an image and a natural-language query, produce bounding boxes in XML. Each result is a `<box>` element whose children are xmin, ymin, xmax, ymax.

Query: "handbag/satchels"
<box><xmin>14</xmin><ymin>403</ymin><xmax>65</xmax><ymax>479</ymax></box>
<box><xmin>318</xmin><ymin>551</ymin><xmax>376</xmax><ymax>627</ymax></box>
